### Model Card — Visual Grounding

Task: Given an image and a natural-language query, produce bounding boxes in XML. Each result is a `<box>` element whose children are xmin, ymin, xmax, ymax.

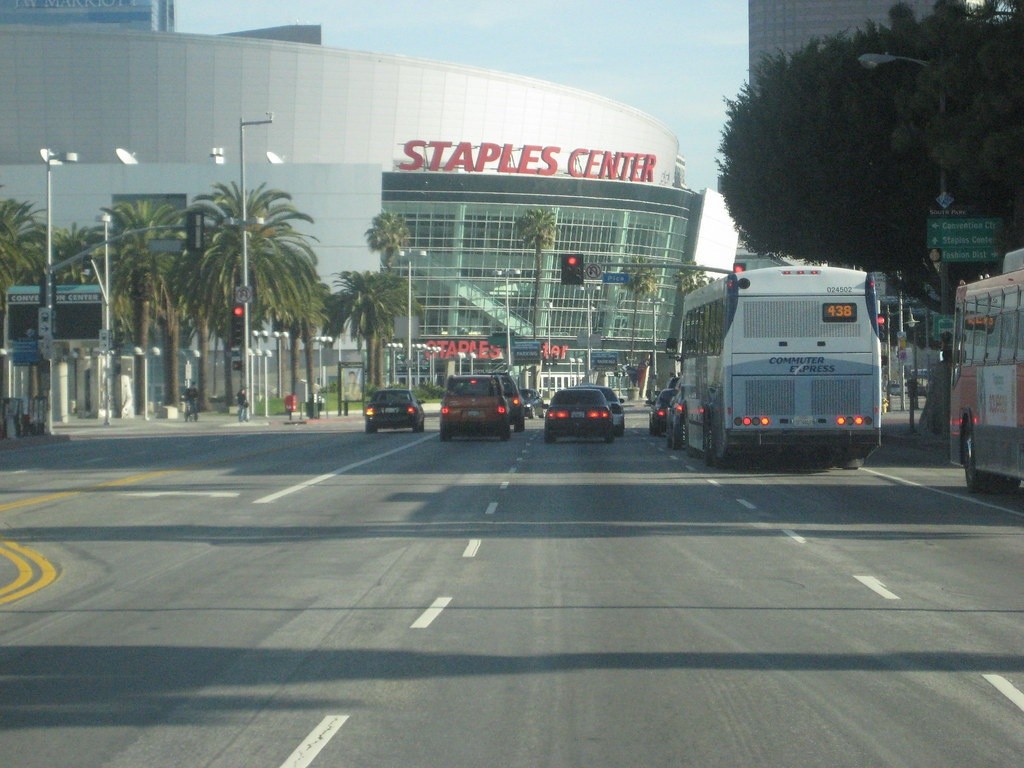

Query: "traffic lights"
<box><xmin>733</xmin><ymin>263</ymin><xmax>746</xmax><ymax>274</ymax></box>
<box><xmin>232</xmin><ymin>304</ymin><xmax>243</xmax><ymax>347</ymax></box>
<box><xmin>561</xmin><ymin>253</ymin><xmax>585</xmax><ymax>285</ymax></box>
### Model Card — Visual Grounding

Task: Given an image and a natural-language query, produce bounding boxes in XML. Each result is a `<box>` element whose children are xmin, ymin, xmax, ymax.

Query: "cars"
<box><xmin>364</xmin><ymin>389</ymin><xmax>425</xmax><ymax>433</ymax></box>
<box><xmin>646</xmin><ymin>377</ymin><xmax>684</xmax><ymax>450</ymax></box>
<box><xmin>542</xmin><ymin>385</ymin><xmax>625</xmax><ymax>444</ymax></box>
<box><xmin>520</xmin><ymin>389</ymin><xmax>545</xmax><ymax>419</ymax></box>
<box><xmin>890</xmin><ymin>382</ymin><xmax>909</xmax><ymax>397</ymax></box>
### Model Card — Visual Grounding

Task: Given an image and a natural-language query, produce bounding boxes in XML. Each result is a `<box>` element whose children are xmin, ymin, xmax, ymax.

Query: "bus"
<box><xmin>950</xmin><ymin>248</ymin><xmax>1024</xmax><ymax>493</ymax></box>
<box><xmin>665</xmin><ymin>266</ymin><xmax>882</xmax><ymax>469</ymax></box>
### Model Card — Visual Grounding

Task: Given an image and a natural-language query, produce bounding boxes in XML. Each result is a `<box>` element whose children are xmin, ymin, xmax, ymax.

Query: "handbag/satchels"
<box><xmin>244</xmin><ymin>402</ymin><xmax>249</xmax><ymax>407</ymax></box>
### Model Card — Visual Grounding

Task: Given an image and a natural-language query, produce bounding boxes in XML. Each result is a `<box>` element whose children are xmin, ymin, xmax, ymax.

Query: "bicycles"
<box><xmin>183</xmin><ymin>397</ymin><xmax>200</xmax><ymax>423</ymax></box>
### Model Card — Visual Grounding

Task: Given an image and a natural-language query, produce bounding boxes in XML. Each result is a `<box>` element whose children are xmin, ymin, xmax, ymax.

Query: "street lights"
<box><xmin>458</xmin><ymin>351</ymin><xmax>466</xmax><ymax>375</ymax></box>
<box><xmin>313</xmin><ymin>336</ymin><xmax>333</xmax><ymax>386</ymax></box>
<box><xmin>133</xmin><ymin>346</ymin><xmax>160</xmax><ymax>423</ymax></box>
<box><xmin>497</xmin><ymin>267</ymin><xmax>521</xmax><ymax>370</ymax></box>
<box><xmin>399</xmin><ymin>248</ymin><xmax>426</xmax><ymax>390</ymax></box>
<box><xmin>44</xmin><ymin>148</ymin><xmax>78</xmax><ymax>436</ymax></box>
<box><xmin>252</xmin><ymin>330</ymin><xmax>289</xmax><ymax>417</ymax></box>
<box><xmin>470</xmin><ymin>353</ymin><xmax>476</xmax><ymax>375</ymax></box>
<box><xmin>896</xmin><ymin>269</ymin><xmax>905</xmax><ymax>411</ymax></box>
<box><xmin>884</xmin><ymin>305</ymin><xmax>920</xmax><ymax>403</ymax></box>
<box><xmin>569</xmin><ymin>357</ymin><xmax>575</xmax><ymax>388</ymax></box>
<box><xmin>648</xmin><ymin>297</ymin><xmax>665</xmax><ymax>375</ymax></box>
<box><xmin>100</xmin><ymin>212</ymin><xmax>112</xmax><ymax>427</ymax></box>
<box><xmin>387</xmin><ymin>343</ymin><xmax>441</xmax><ymax>385</ymax></box>
<box><xmin>581</xmin><ymin>285</ymin><xmax>601</xmax><ymax>373</ymax></box>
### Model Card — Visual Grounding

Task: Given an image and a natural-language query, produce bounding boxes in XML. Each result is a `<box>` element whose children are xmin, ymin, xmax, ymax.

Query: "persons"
<box><xmin>181</xmin><ymin>382</ymin><xmax>199</xmax><ymax>422</ymax></box>
<box><xmin>237</xmin><ymin>388</ymin><xmax>246</xmax><ymax>421</ymax></box>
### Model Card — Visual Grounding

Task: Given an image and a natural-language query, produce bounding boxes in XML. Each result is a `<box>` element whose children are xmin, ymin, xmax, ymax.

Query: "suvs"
<box><xmin>440</xmin><ymin>371</ymin><xmax>526</xmax><ymax>441</ymax></box>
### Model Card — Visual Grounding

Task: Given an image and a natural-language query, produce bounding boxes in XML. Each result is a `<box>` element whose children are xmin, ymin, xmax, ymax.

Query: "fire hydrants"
<box><xmin>882</xmin><ymin>398</ymin><xmax>889</xmax><ymax>414</ymax></box>
